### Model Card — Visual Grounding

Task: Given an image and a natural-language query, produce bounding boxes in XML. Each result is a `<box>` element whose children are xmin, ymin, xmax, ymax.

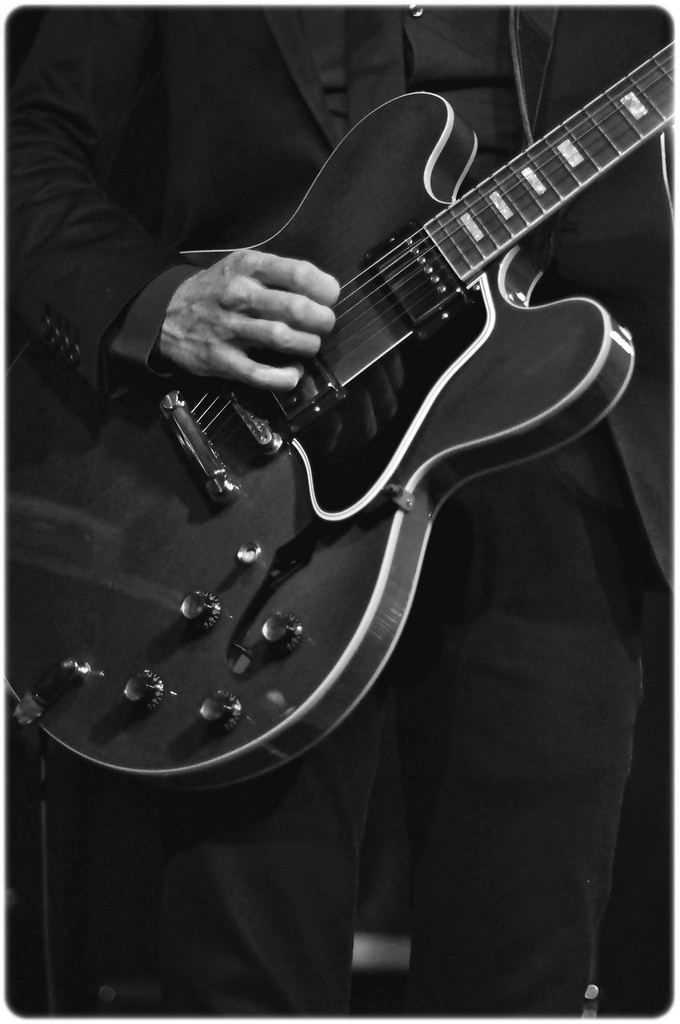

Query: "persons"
<box><xmin>6</xmin><ymin>6</ymin><xmax>674</xmax><ymax>1017</ymax></box>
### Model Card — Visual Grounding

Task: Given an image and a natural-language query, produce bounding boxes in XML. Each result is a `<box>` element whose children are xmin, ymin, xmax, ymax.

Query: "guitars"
<box><xmin>4</xmin><ymin>31</ymin><xmax>675</xmax><ymax>803</ymax></box>
